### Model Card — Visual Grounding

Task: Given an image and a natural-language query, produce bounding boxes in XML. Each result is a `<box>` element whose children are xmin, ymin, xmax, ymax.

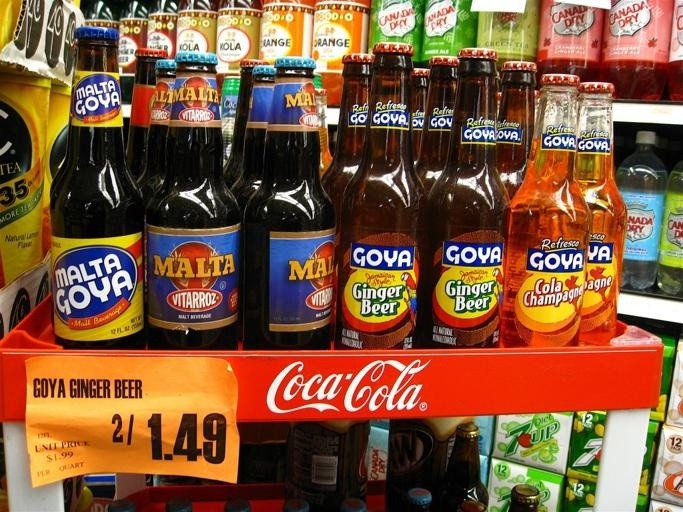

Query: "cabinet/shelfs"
<box><xmin>599</xmin><ymin>91</ymin><xmax>682</xmax><ymax>325</ymax></box>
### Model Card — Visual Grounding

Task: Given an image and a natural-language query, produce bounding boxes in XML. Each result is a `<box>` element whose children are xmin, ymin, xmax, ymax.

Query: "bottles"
<box><xmin>610</xmin><ymin>123</ymin><xmax>683</xmax><ymax>301</ymax></box>
<box><xmin>111</xmin><ymin>414</ymin><xmax>540</xmax><ymax>511</ymax></box>
<box><xmin>49</xmin><ymin>25</ymin><xmax>627</xmax><ymax>348</ymax></box>
<box><xmin>81</xmin><ymin>1</ymin><xmax>682</xmax><ymax>105</ymax></box>
